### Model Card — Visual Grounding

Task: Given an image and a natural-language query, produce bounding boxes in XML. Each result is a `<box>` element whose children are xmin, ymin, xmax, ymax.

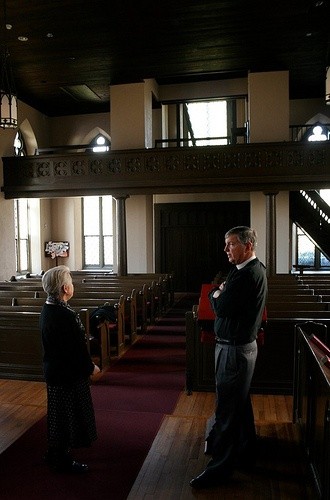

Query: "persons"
<box><xmin>40</xmin><ymin>265</ymin><xmax>102</xmax><ymax>475</ymax></box>
<box><xmin>189</xmin><ymin>227</ymin><xmax>268</xmax><ymax>490</ymax></box>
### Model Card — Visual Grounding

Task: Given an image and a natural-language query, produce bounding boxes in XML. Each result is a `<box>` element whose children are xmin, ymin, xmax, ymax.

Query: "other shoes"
<box><xmin>53</xmin><ymin>460</ymin><xmax>89</xmax><ymax>475</ymax></box>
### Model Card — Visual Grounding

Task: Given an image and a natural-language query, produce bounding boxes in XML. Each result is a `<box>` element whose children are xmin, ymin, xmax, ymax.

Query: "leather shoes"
<box><xmin>188</xmin><ymin>469</ymin><xmax>232</xmax><ymax>490</ymax></box>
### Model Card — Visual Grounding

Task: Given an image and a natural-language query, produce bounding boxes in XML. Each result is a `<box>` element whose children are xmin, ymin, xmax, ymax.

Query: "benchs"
<box><xmin>0</xmin><ymin>268</ymin><xmax>173</xmax><ymax>384</ymax></box>
<box><xmin>184</xmin><ymin>262</ymin><xmax>330</xmax><ymax>497</ymax></box>
